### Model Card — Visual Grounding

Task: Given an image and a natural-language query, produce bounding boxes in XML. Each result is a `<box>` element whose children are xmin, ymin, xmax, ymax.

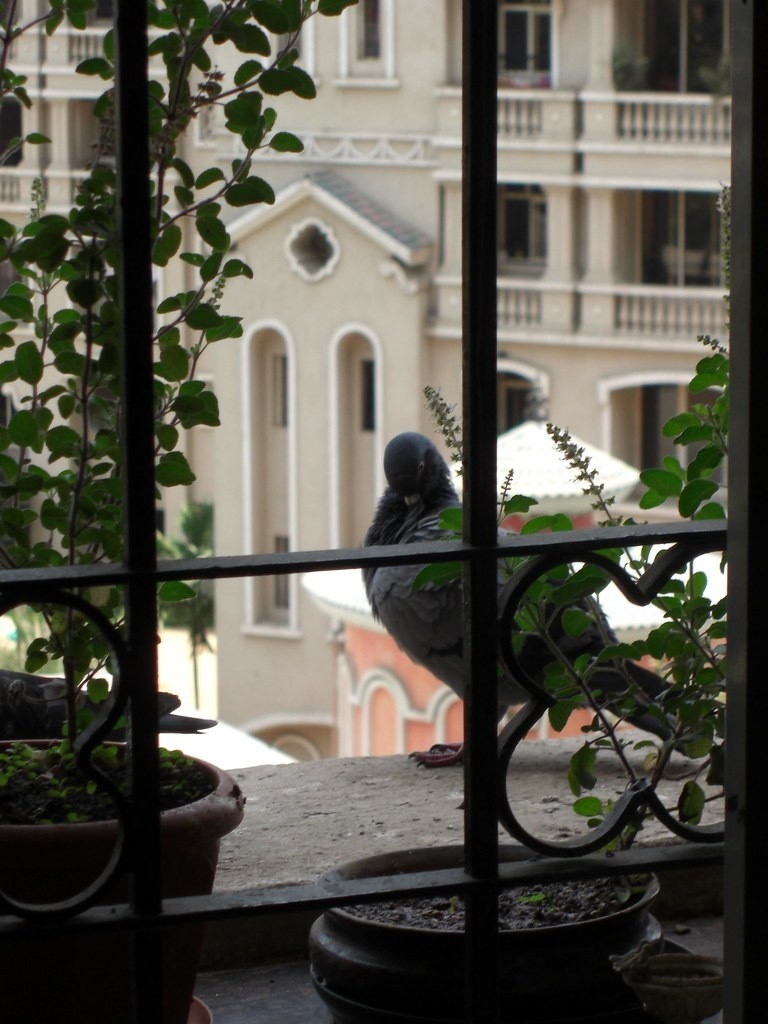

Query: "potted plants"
<box><xmin>0</xmin><ymin>0</ymin><xmax>364</xmax><ymax>1024</ymax></box>
<box><xmin>308</xmin><ymin>186</ymin><xmax>732</xmax><ymax>1024</ymax></box>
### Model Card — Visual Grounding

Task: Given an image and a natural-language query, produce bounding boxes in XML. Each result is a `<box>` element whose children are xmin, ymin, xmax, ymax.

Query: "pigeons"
<box><xmin>361</xmin><ymin>433</ymin><xmax>707</xmax><ymax>768</ymax></box>
<box><xmin>2</xmin><ymin>667</ymin><xmax>216</xmax><ymax>746</ymax></box>
